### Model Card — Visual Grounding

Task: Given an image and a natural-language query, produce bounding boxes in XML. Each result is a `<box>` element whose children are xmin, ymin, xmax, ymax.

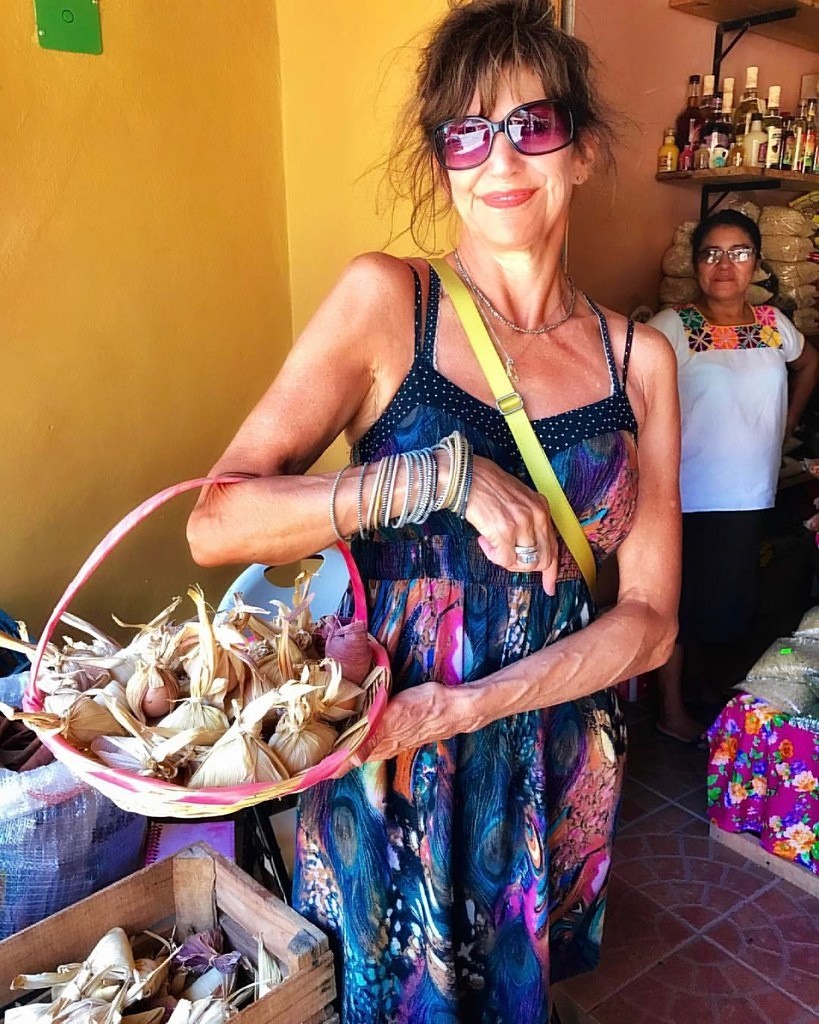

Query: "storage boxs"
<box><xmin>1</xmin><ymin>841</ymin><xmax>341</xmax><ymax>1024</ymax></box>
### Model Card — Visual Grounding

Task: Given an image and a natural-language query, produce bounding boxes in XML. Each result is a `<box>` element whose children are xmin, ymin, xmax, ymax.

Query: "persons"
<box><xmin>181</xmin><ymin>1</ymin><xmax>686</xmax><ymax>1021</ymax></box>
<box><xmin>633</xmin><ymin>208</ymin><xmax>819</xmax><ymax>751</ymax></box>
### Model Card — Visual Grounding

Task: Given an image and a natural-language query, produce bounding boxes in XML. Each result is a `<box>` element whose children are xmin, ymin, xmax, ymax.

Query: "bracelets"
<box><xmin>329</xmin><ymin>428</ymin><xmax>476</xmax><ymax>542</ymax></box>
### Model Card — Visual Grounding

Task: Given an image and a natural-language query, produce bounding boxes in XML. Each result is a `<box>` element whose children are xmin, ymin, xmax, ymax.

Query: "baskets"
<box><xmin>21</xmin><ymin>471</ymin><xmax>390</xmax><ymax>818</ymax></box>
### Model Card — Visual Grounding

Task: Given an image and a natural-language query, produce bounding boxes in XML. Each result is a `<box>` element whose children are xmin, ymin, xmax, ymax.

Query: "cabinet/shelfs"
<box><xmin>656</xmin><ymin>0</ymin><xmax>819</xmax><ymax>218</ymax></box>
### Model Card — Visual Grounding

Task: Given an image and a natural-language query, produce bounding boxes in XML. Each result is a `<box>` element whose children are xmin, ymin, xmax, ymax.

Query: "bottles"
<box><xmin>656</xmin><ymin>67</ymin><xmax>819</xmax><ymax>176</ymax></box>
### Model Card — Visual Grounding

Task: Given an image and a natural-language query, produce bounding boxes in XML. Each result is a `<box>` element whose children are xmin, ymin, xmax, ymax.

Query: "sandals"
<box><xmin>652</xmin><ymin>721</ymin><xmax>712</xmax><ymax>753</ymax></box>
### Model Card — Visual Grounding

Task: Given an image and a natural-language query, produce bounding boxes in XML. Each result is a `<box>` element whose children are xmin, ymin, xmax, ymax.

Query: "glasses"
<box><xmin>696</xmin><ymin>247</ymin><xmax>757</xmax><ymax>264</ymax></box>
<box><xmin>431</xmin><ymin>99</ymin><xmax>575</xmax><ymax>170</ymax></box>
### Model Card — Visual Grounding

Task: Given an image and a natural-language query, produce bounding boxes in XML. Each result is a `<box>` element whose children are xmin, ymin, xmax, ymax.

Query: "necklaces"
<box><xmin>453</xmin><ymin>247</ymin><xmax>575</xmax><ymax>380</ymax></box>
